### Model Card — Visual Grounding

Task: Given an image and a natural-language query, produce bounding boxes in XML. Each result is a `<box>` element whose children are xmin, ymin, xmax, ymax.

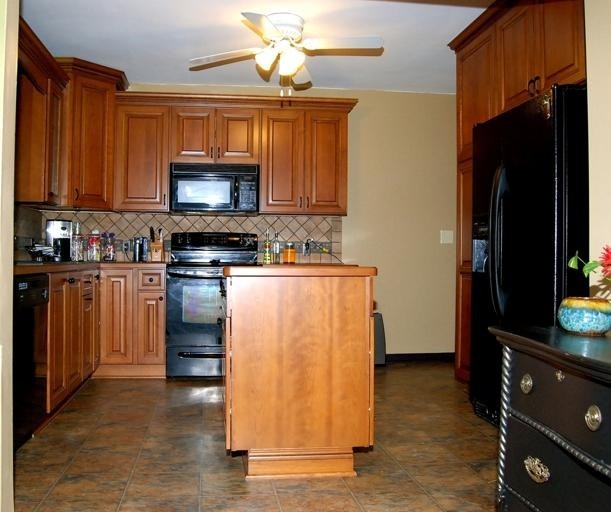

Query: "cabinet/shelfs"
<box><xmin>221</xmin><ymin>264</ymin><xmax>379</xmax><ymax>482</ymax></box>
<box><xmin>497</xmin><ymin>0</ymin><xmax>587</xmax><ymax>112</ymax></box>
<box><xmin>112</xmin><ymin>91</ymin><xmax>170</xmax><ymax>211</ymax></box>
<box><xmin>137</xmin><ymin>264</ymin><xmax>166</xmax><ymax>379</ymax></box>
<box><xmin>80</xmin><ymin>265</ymin><xmax>93</xmax><ymax>387</ymax></box>
<box><xmin>259</xmin><ymin>95</ymin><xmax>358</xmax><ymax>215</ymax></box>
<box><xmin>171</xmin><ymin>93</ymin><xmax>259</xmax><ymax>163</ymax></box>
<box><xmin>486</xmin><ymin>324</ymin><xmax>609</xmax><ymax>512</ymax></box>
<box><xmin>56</xmin><ymin>56</ymin><xmax>129</xmax><ymax>209</ymax></box>
<box><xmin>90</xmin><ymin>264</ymin><xmax>101</xmax><ymax>378</ymax></box>
<box><xmin>446</xmin><ymin>0</ymin><xmax>503</xmax><ymax>385</ymax></box>
<box><xmin>19</xmin><ymin>14</ymin><xmax>69</xmax><ymax>203</ymax></box>
<box><xmin>99</xmin><ymin>264</ymin><xmax>133</xmax><ymax>380</ymax></box>
<box><xmin>48</xmin><ymin>266</ymin><xmax>82</xmax><ymax>421</ymax></box>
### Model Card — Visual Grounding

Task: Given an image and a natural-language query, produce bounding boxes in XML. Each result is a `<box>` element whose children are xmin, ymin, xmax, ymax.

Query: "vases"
<box><xmin>558</xmin><ymin>297</ymin><xmax>610</xmax><ymax>335</ymax></box>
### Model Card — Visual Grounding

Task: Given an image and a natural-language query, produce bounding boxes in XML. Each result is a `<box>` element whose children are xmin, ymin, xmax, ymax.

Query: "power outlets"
<box><xmin>303</xmin><ymin>244</ymin><xmax>311</xmax><ymax>256</ymax></box>
<box><xmin>123</xmin><ymin>241</ymin><xmax>130</xmax><ymax>253</ymax></box>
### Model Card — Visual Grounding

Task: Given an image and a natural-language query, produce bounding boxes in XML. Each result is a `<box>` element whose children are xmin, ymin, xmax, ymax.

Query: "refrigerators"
<box><xmin>471</xmin><ymin>84</ymin><xmax>588</xmax><ymax>428</ymax></box>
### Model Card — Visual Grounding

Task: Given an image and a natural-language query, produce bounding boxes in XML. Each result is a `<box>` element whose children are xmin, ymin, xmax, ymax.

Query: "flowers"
<box><xmin>566</xmin><ymin>244</ymin><xmax>611</xmax><ymax>300</ymax></box>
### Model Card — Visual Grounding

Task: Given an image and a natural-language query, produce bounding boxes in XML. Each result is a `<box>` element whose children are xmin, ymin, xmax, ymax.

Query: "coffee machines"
<box><xmin>46</xmin><ymin>218</ymin><xmax>72</xmax><ymax>262</ymax></box>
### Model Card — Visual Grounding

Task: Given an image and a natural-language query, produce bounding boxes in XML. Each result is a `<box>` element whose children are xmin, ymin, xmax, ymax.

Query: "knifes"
<box><xmin>150</xmin><ymin>226</ymin><xmax>163</xmax><ymax>243</ymax></box>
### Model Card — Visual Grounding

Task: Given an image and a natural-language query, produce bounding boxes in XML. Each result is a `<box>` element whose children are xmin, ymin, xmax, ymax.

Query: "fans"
<box><xmin>190</xmin><ymin>12</ymin><xmax>385</xmax><ymax>90</ymax></box>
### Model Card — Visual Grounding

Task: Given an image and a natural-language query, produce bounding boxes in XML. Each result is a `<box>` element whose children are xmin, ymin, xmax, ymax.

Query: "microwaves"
<box><xmin>170</xmin><ymin>162</ymin><xmax>259</xmax><ymax>217</ymax></box>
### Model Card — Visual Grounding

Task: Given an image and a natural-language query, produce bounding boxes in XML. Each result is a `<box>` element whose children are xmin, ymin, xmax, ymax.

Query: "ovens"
<box><xmin>166</xmin><ymin>267</ymin><xmax>227</xmax><ymax>380</ymax></box>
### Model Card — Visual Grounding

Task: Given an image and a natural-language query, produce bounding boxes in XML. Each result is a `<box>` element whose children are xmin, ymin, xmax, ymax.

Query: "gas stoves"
<box><xmin>166</xmin><ymin>232</ymin><xmax>263</xmax><ymax>267</ymax></box>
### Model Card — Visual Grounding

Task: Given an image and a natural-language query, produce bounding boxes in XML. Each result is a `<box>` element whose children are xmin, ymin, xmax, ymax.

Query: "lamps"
<box><xmin>254</xmin><ymin>39</ymin><xmax>306</xmax><ymax>76</ymax></box>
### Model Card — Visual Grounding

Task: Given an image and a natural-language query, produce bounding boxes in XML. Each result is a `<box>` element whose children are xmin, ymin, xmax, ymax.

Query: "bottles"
<box><xmin>272</xmin><ymin>230</ymin><xmax>280</xmax><ymax>264</ymax></box>
<box><xmin>263</xmin><ymin>227</ymin><xmax>271</xmax><ymax>264</ymax></box>
<box><xmin>72</xmin><ymin>222</ymin><xmax>147</xmax><ymax>263</ymax></box>
<box><xmin>283</xmin><ymin>242</ymin><xmax>296</xmax><ymax>264</ymax></box>
